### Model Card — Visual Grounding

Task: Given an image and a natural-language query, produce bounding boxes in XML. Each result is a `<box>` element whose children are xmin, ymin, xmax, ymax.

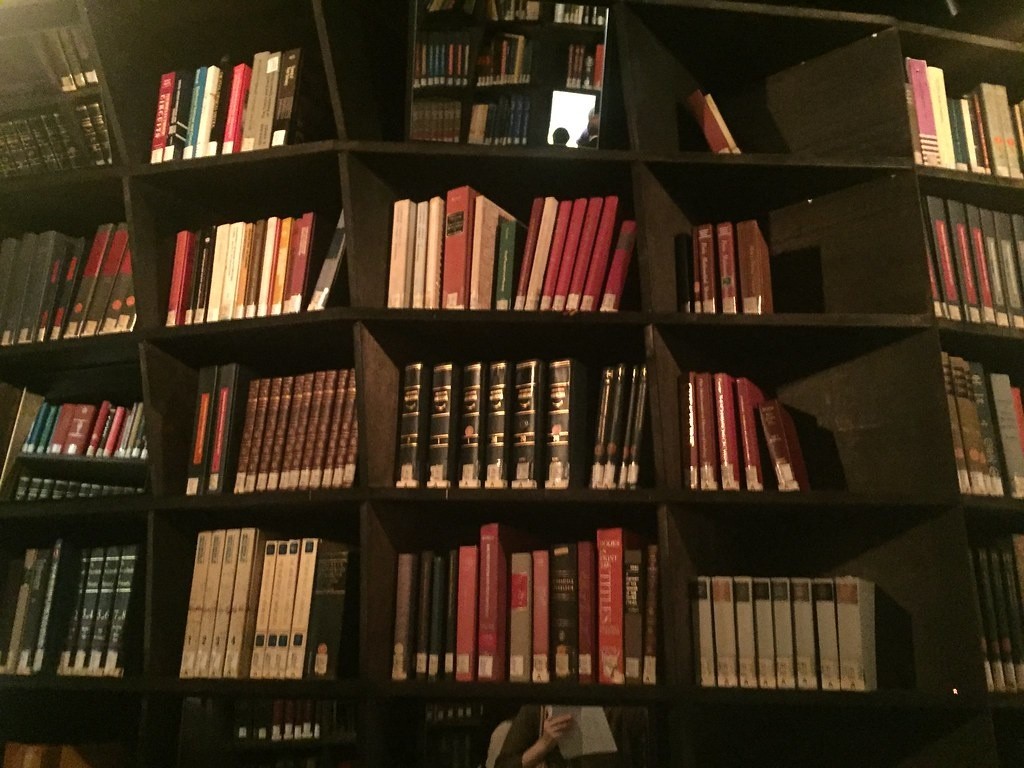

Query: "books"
<box><xmin>0</xmin><ymin>1</ymin><xmax>1024</xmax><ymax>768</ymax></box>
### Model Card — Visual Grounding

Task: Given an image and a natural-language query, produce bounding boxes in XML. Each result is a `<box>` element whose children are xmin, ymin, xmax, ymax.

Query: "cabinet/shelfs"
<box><xmin>0</xmin><ymin>0</ymin><xmax>178</xmax><ymax>768</ymax></box>
<box><xmin>82</xmin><ymin>0</ymin><xmax>401</xmax><ymax>768</ymax></box>
<box><xmin>901</xmin><ymin>24</ymin><xmax>1024</xmax><ymax>768</ymax></box>
<box><xmin>312</xmin><ymin>3</ymin><xmax>705</xmax><ymax>768</ymax></box>
<box><xmin>616</xmin><ymin>0</ymin><xmax>993</xmax><ymax>768</ymax></box>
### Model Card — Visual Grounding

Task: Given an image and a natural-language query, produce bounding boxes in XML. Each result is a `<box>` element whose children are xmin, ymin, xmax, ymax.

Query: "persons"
<box><xmin>494</xmin><ymin>701</ymin><xmax>649</xmax><ymax>768</ymax></box>
